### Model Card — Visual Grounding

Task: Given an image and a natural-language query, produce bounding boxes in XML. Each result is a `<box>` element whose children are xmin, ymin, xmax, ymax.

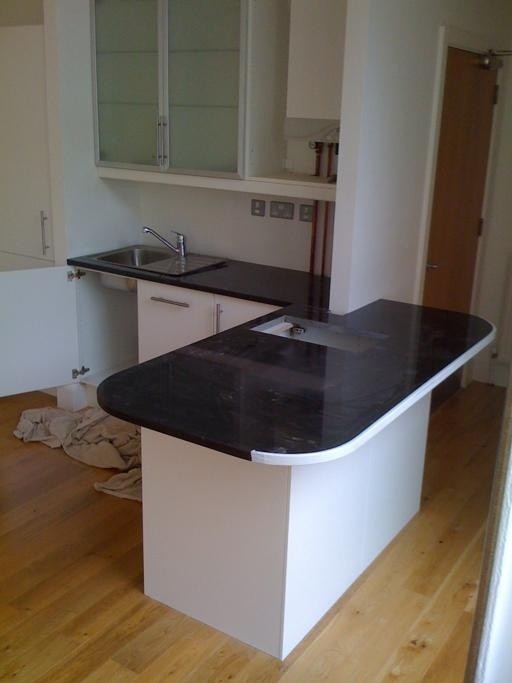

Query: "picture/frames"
<box><xmin>90</xmin><ymin>1</ymin><xmax>290</xmax><ymax>180</ymax></box>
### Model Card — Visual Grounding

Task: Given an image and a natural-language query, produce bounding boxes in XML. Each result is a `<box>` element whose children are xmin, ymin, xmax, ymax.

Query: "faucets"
<box><xmin>141</xmin><ymin>226</ymin><xmax>180</xmax><ymax>253</ymax></box>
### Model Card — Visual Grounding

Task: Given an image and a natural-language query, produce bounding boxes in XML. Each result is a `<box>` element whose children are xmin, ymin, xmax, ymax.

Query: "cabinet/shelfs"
<box><xmin>0</xmin><ymin>267</ymin><xmax>284</xmax><ymax>398</ymax></box>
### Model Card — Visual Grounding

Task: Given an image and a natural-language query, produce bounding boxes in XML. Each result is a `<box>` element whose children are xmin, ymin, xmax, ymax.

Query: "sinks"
<box><xmin>95</xmin><ymin>245</ymin><xmax>189</xmax><ymax>267</ymax></box>
